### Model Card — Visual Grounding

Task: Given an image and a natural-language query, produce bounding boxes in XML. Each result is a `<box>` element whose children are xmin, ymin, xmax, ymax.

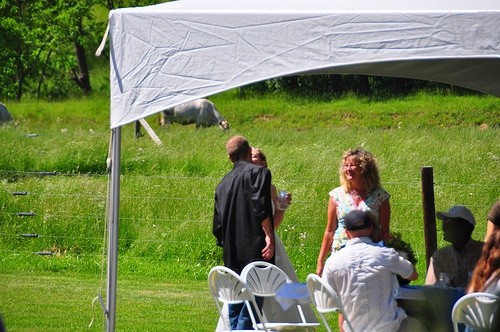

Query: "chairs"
<box><xmin>208</xmin><ymin>261</ymin><xmax>500</xmax><ymax>332</ymax></box>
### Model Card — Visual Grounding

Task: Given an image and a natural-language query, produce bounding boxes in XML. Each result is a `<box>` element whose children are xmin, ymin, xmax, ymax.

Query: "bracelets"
<box><xmin>279</xmin><ymin>208</ymin><xmax>286</xmax><ymax>210</ymax></box>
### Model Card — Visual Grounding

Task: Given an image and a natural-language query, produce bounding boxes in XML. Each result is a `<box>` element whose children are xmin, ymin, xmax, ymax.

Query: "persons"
<box><xmin>320</xmin><ymin>210</ymin><xmax>418</xmax><ymax>332</ymax></box>
<box><xmin>211</xmin><ymin>135</ymin><xmax>276</xmax><ymax>330</ymax></box>
<box><xmin>315</xmin><ymin>148</ymin><xmax>393</xmax><ymax>332</ymax></box>
<box><xmin>215</xmin><ymin>147</ymin><xmax>319</xmax><ymax>332</ymax></box>
<box><xmin>422</xmin><ymin>201</ymin><xmax>500</xmax><ymax>332</ymax></box>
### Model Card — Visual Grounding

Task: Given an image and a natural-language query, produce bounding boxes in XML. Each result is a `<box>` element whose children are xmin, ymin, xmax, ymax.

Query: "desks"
<box><xmin>394</xmin><ymin>286</ymin><xmax>468</xmax><ymax>332</ymax></box>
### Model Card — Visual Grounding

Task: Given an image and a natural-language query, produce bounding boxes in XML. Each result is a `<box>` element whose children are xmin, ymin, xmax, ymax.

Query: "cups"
<box><xmin>278</xmin><ymin>192</ymin><xmax>288</xmax><ymax>210</ymax></box>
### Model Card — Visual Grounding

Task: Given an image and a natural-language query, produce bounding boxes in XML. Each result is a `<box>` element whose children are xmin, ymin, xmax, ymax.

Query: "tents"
<box><xmin>95</xmin><ymin>0</ymin><xmax>500</xmax><ymax>332</ymax></box>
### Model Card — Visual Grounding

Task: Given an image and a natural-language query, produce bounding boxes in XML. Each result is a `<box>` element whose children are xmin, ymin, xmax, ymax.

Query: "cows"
<box><xmin>159</xmin><ymin>97</ymin><xmax>230</xmax><ymax>132</ymax></box>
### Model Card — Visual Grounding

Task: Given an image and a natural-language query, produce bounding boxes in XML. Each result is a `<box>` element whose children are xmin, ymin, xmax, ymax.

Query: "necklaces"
<box><xmin>351</xmin><ymin>190</ymin><xmax>362</xmax><ymax>199</ymax></box>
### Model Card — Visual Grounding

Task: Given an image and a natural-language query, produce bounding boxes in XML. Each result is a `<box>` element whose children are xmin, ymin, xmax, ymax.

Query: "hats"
<box><xmin>436</xmin><ymin>206</ymin><xmax>475</xmax><ymax>226</ymax></box>
<box><xmin>345</xmin><ymin>211</ymin><xmax>372</xmax><ymax>231</ymax></box>
